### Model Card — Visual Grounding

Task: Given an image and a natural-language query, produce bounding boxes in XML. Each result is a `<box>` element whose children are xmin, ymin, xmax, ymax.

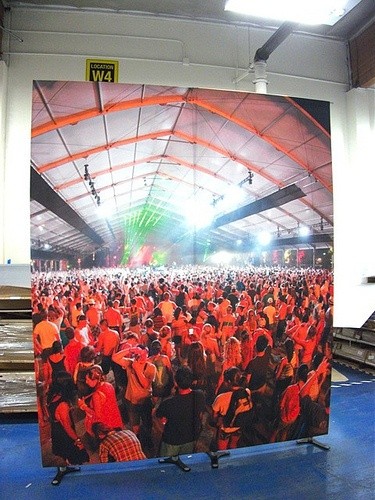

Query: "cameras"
<box><xmin>134</xmin><ymin>353</ymin><xmax>140</xmax><ymax>361</ymax></box>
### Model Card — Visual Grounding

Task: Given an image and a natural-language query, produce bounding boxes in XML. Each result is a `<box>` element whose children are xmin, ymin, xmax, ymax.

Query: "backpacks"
<box><xmin>220</xmin><ymin>387</ymin><xmax>255</xmax><ymax>431</ymax></box>
<box><xmin>307</xmin><ymin>400</ymin><xmax>323</xmax><ymax>427</ymax></box>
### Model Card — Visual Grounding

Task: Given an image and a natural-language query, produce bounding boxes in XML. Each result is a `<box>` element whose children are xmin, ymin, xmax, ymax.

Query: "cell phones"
<box><xmin>188</xmin><ymin>328</ymin><xmax>194</xmax><ymax>338</ymax></box>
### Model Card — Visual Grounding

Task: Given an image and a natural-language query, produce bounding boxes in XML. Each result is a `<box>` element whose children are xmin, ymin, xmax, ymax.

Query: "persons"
<box><xmin>32</xmin><ymin>265</ymin><xmax>335</xmax><ymax>466</ymax></box>
